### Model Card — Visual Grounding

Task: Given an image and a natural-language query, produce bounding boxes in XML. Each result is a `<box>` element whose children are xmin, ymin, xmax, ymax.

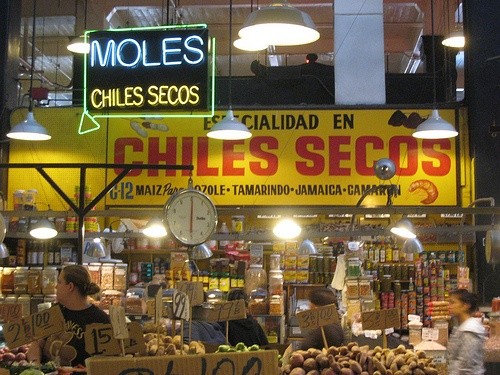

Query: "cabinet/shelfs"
<box><xmin>0</xmin><ymin>231</ymin><xmax>465</xmax><ymax>343</ymax></box>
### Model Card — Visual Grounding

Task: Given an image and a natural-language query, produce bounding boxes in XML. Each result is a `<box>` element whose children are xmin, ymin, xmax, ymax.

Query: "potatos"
<box><xmin>0</xmin><ymin>343</ymin><xmax>32</xmax><ymax>367</ymax></box>
<box><xmin>281</xmin><ymin>342</ymin><xmax>438</xmax><ymax>375</ymax></box>
<box><xmin>123</xmin><ymin>333</ymin><xmax>206</xmax><ymax>358</ymax></box>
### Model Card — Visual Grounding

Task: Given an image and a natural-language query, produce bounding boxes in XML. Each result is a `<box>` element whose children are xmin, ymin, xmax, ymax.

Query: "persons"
<box><xmin>441</xmin><ymin>288</ymin><xmax>488</xmax><ymax>375</ymax></box>
<box><xmin>21</xmin><ymin>263</ymin><xmax>115</xmax><ymax>375</ymax></box>
<box><xmin>218</xmin><ymin>288</ymin><xmax>271</xmax><ymax>349</ymax></box>
<box><xmin>177</xmin><ymin>289</ymin><xmax>234</xmax><ymax>353</ymax></box>
<box><xmin>291</xmin><ymin>285</ymin><xmax>347</xmax><ymax>357</ymax></box>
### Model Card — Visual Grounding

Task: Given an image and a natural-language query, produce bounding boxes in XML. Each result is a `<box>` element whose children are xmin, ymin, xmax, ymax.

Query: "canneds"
<box><xmin>65</xmin><ymin>185</ymin><xmax>97</xmax><ymax>232</ymax></box>
<box><xmin>309</xmin><ymin>254</ymin><xmax>336</xmax><ymax>284</ymax></box>
<box><xmin>380</xmin><ymin>267</ymin><xmax>450</xmax><ymax>329</ymax></box>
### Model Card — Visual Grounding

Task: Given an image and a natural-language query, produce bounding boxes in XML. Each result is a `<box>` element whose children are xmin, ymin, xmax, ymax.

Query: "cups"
<box><xmin>423</xmin><ymin>250</ymin><xmax>465</xmax><ymax>263</ymax></box>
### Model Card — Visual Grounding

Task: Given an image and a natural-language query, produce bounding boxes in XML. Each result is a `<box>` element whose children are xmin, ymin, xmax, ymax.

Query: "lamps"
<box><xmin>67</xmin><ymin>0</ymin><xmax>90</xmax><ymax>54</ymax></box>
<box><xmin>192</xmin><ymin>243</ymin><xmax>213</xmax><ymax>280</ymax></box>
<box><xmin>401</xmin><ymin>237</ymin><xmax>424</xmax><ymax>254</ymax></box>
<box><xmin>298</xmin><ymin>238</ymin><xmax>318</xmax><ymax>255</ymax></box>
<box><xmin>375</xmin><ymin>158</ymin><xmax>395</xmax><ymax>185</ymax></box>
<box><xmin>84</xmin><ymin>237</ymin><xmax>106</xmax><ymax>258</ymax></box>
<box><xmin>143</xmin><ymin>216</ymin><xmax>167</xmax><ymax>238</ymax></box>
<box><xmin>238</xmin><ymin>0</ymin><xmax>320</xmax><ymax>45</ymax></box>
<box><xmin>6</xmin><ymin>0</ymin><xmax>51</xmax><ymax>141</ymax></box>
<box><xmin>30</xmin><ymin>216</ymin><xmax>58</xmax><ymax>239</ymax></box>
<box><xmin>442</xmin><ymin>0</ymin><xmax>465</xmax><ymax>49</ymax></box>
<box><xmin>207</xmin><ymin>0</ymin><xmax>252</xmax><ymax>141</ymax></box>
<box><xmin>0</xmin><ymin>243</ymin><xmax>10</xmax><ymax>259</ymax></box>
<box><xmin>412</xmin><ymin>0</ymin><xmax>458</xmax><ymax>139</ymax></box>
<box><xmin>392</xmin><ymin>213</ymin><xmax>417</xmax><ymax>239</ymax></box>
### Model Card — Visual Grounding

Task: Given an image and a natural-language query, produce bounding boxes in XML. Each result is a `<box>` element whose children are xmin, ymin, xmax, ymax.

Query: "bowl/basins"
<box><xmin>7</xmin><ymin>240</ymin><xmax>26</xmax><ymax>267</ymax></box>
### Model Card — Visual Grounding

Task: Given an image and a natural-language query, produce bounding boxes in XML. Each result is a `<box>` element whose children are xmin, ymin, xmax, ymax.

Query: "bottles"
<box><xmin>193</xmin><ymin>254</ymin><xmax>288</xmax><ymax>317</ymax></box>
<box><xmin>308</xmin><ymin>237</ymin><xmax>399</xmax><ymax>312</ymax></box>
<box><xmin>219</xmin><ymin>222</ymin><xmax>230</xmax><ymax>250</ymax></box>
<box><xmin>24</xmin><ymin>239</ymin><xmax>61</xmax><ymax>265</ymax></box>
<box><xmin>231</xmin><ymin>215</ymin><xmax>247</xmax><ymax>234</ymax></box>
<box><xmin>0</xmin><ymin>262</ymin><xmax>129</xmax><ymax>295</ymax></box>
<box><xmin>435</xmin><ymin>319</ymin><xmax>449</xmax><ymax>346</ymax></box>
